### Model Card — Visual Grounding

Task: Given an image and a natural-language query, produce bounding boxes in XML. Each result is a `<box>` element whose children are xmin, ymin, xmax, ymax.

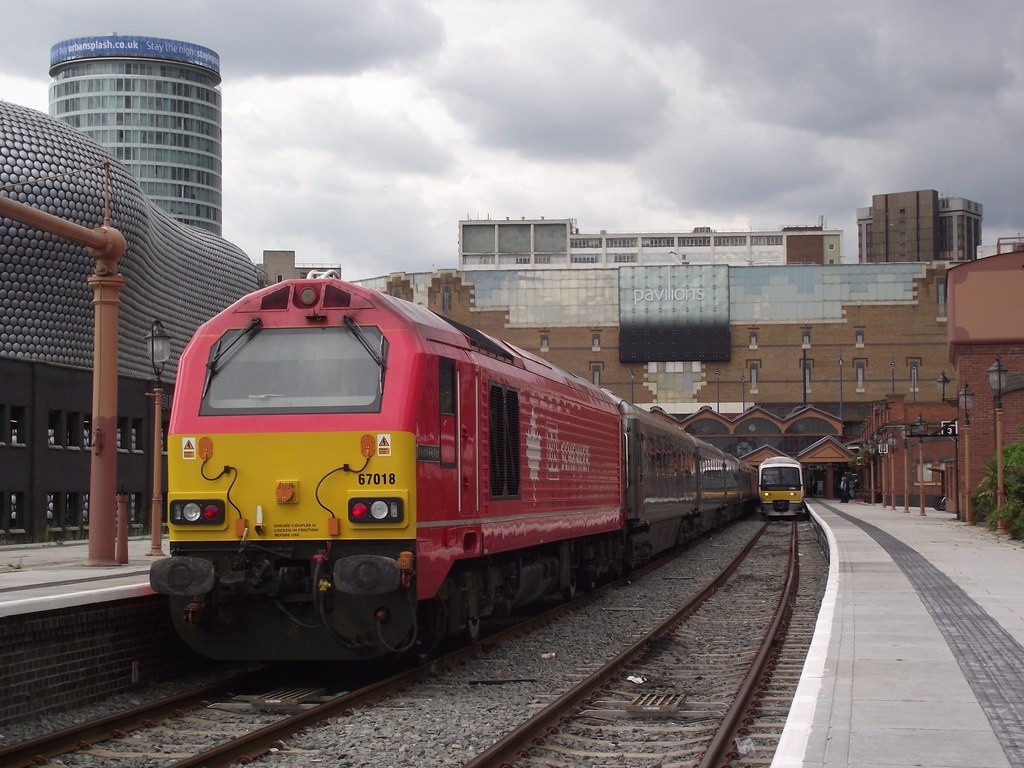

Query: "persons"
<box><xmin>812</xmin><ymin>480</ymin><xmax>818</xmax><ymax>497</ymax></box>
<box><xmin>838</xmin><ymin>476</ymin><xmax>849</xmax><ymax>503</ymax></box>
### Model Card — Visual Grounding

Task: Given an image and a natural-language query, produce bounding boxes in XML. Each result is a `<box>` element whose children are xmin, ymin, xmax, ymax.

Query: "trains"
<box><xmin>755</xmin><ymin>457</ymin><xmax>806</xmax><ymax>518</ymax></box>
<box><xmin>150</xmin><ymin>270</ymin><xmax>761</xmax><ymax>664</ymax></box>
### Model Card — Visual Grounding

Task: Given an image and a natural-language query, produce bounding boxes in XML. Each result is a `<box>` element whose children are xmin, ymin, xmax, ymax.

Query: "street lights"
<box><xmin>888</xmin><ymin>431</ymin><xmax>899</xmax><ymax>510</ymax></box>
<box><xmin>958</xmin><ymin>383</ymin><xmax>980</xmax><ymax>526</ymax></box>
<box><xmin>879</xmin><ymin>438</ymin><xmax>887</xmax><ymax>509</ymax></box>
<box><xmin>900</xmin><ymin>424</ymin><xmax>910</xmax><ymax>513</ymax></box>
<box><xmin>144</xmin><ymin>318</ymin><xmax>172</xmax><ymax>557</ymax></box>
<box><xmin>914</xmin><ymin>414</ymin><xmax>928</xmax><ymax>516</ymax></box>
<box><xmin>868</xmin><ymin>444</ymin><xmax>877</xmax><ymax>506</ymax></box>
<box><xmin>986</xmin><ymin>356</ymin><xmax>1011</xmax><ymax>535</ymax></box>
